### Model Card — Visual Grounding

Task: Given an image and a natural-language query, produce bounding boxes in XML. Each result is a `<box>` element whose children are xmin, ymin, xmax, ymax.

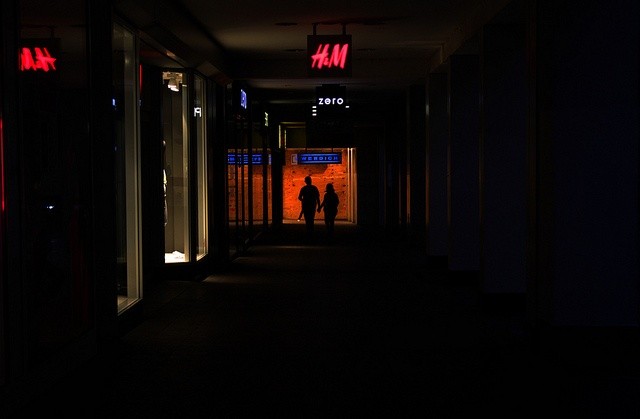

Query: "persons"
<box><xmin>317</xmin><ymin>183</ymin><xmax>339</xmax><ymax>237</ymax></box>
<box><xmin>298</xmin><ymin>175</ymin><xmax>321</xmax><ymax>233</ymax></box>
